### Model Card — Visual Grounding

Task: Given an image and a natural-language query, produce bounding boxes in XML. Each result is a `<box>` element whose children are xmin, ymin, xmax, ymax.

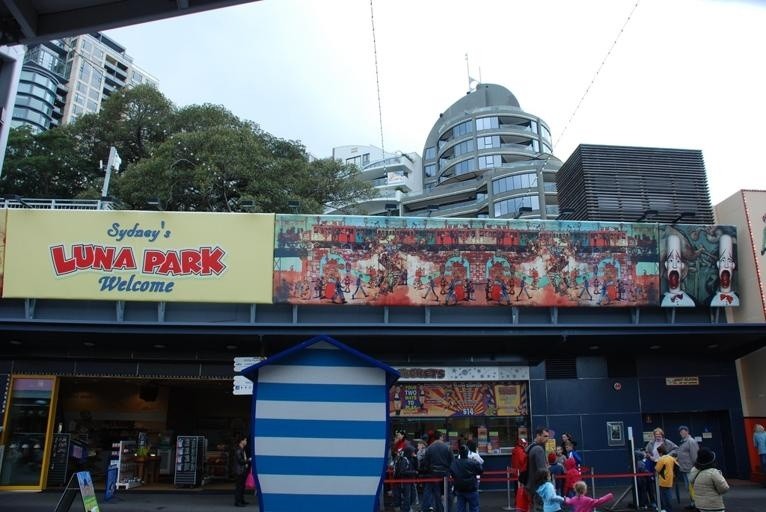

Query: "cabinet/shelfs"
<box><xmin>51</xmin><ymin>430</ymin><xmax>231</xmax><ymax>491</ymax></box>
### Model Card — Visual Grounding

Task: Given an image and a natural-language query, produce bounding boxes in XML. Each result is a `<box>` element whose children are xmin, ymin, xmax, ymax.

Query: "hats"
<box><xmin>694</xmin><ymin>447</ymin><xmax>718</xmax><ymax>470</ymax></box>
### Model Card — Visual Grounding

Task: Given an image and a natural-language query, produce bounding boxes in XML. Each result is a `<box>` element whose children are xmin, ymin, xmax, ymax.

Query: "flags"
<box><xmin>110</xmin><ymin>149</ymin><xmax>122</xmax><ymax>171</ymax></box>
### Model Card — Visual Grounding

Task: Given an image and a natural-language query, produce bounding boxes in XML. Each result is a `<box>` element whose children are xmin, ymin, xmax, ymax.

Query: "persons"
<box><xmin>231</xmin><ymin>435</ymin><xmax>252</xmax><ymax>508</ymax></box>
<box><xmin>384</xmin><ymin>422</ymin><xmax>766</xmax><ymax>512</ymax></box>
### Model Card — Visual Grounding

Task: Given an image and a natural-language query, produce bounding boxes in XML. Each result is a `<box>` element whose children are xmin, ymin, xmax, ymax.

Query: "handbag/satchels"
<box><xmin>233</xmin><ymin>459</ymin><xmax>244</xmax><ymax>475</ymax></box>
<box><xmin>244</xmin><ymin>473</ymin><xmax>256</xmax><ymax>490</ymax></box>
